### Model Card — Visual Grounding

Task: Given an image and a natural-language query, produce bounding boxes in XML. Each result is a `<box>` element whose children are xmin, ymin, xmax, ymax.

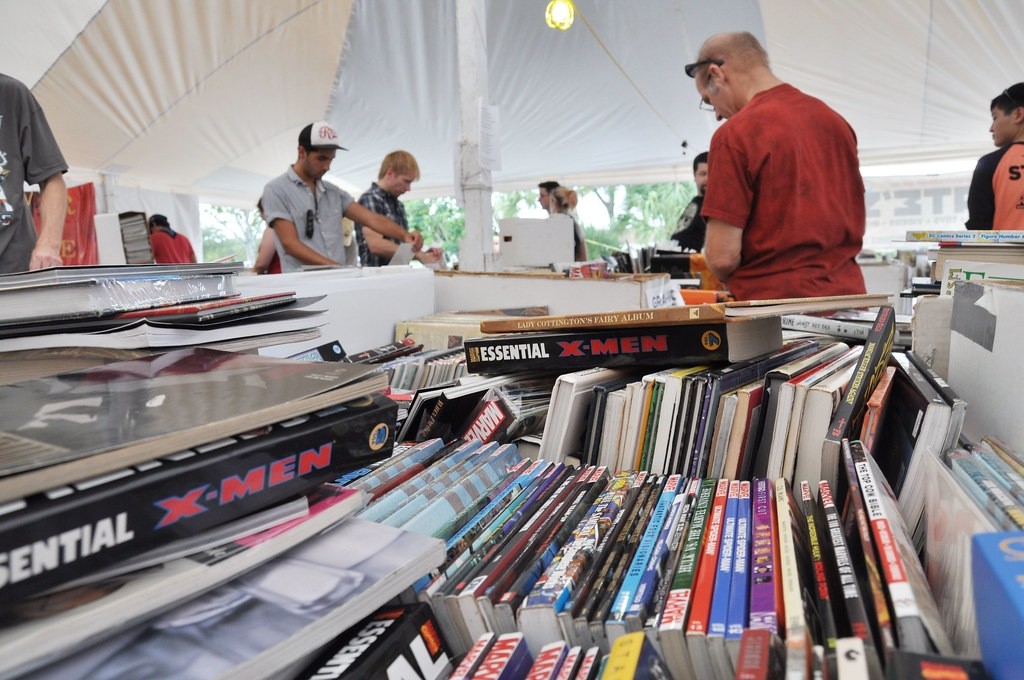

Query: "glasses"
<box><xmin>306</xmin><ymin>209</ymin><xmax>314</xmax><ymax>238</ymax></box>
<box><xmin>685</xmin><ymin>58</ymin><xmax>726</xmax><ymax>79</ymax></box>
<box><xmin>1001</xmin><ymin>89</ymin><xmax>1020</xmax><ymax>109</ymax></box>
<box><xmin>698</xmin><ymin>73</ymin><xmax>716</xmax><ymax>111</ymax></box>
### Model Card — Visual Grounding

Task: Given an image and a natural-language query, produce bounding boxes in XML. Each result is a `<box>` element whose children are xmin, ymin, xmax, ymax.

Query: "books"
<box><xmin>0</xmin><ymin>230</ymin><xmax>1023</xmax><ymax>680</ymax></box>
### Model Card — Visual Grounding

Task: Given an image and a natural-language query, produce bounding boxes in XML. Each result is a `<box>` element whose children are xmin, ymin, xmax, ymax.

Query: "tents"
<box><xmin>0</xmin><ymin>0</ymin><xmax>1023</xmax><ymax>276</ymax></box>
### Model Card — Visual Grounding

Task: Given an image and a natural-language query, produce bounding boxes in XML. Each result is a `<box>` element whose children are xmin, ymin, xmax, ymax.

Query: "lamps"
<box><xmin>545</xmin><ymin>0</ymin><xmax>574</xmax><ymax>30</ymax></box>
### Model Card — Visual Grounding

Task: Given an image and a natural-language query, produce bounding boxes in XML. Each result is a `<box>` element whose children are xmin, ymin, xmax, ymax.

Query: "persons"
<box><xmin>144</xmin><ymin>214</ymin><xmax>195</xmax><ymax>263</ymax></box>
<box><xmin>672</xmin><ymin>152</ymin><xmax>710</xmax><ymax>252</ymax></box>
<box><xmin>355</xmin><ymin>151</ymin><xmax>444</xmax><ymax>266</ymax></box>
<box><xmin>260</xmin><ymin>122</ymin><xmax>423</xmax><ymax>268</ymax></box>
<box><xmin>253</xmin><ymin>198</ymin><xmax>282</xmax><ymax>274</ymax></box>
<box><xmin>537</xmin><ymin>181</ymin><xmax>563</xmax><ymax>216</ymax></box>
<box><xmin>550</xmin><ymin>187</ymin><xmax>587</xmax><ymax>261</ymax></box>
<box><xmin>685</xmin><ymin>31</ymin><xmax>870</xmax><ymax>310</ymax></box>
<box><xmin>0</xmin><ymin>74</ymin><xmax>69</xmax><ymax>275</ymax></box>
<box><xmin>964</xmin><ymin>81</ymin><xmax>1024</xmax><ymax>229</ymax></box>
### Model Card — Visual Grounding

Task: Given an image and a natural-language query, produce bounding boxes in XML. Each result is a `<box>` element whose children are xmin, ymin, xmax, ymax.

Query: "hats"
<box><xmin>299</xmin><ymin>122</ymin><xmax>348</xmax><ymax>151</ymax></box>
<box><xmin>148</xmin><ymin>214</ymin><xmax>168</xmax><ymax>224</ymax></box>
